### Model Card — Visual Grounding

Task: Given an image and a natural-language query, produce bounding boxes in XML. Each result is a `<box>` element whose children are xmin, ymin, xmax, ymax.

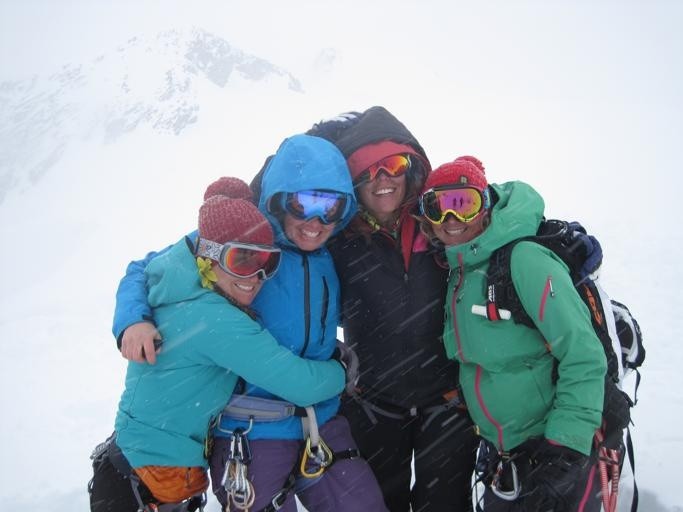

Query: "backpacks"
<box><xmin>486</xmin><ymin>218</ymin><xmax>645</xmax><ymax>427</ymax></box>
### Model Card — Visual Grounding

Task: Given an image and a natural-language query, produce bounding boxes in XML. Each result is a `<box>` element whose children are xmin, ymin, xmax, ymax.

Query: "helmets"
<box><xmin>422</xmin><ymin>187</ymin><xmax>491</xmax><ymax>223</ymax></box>
<box><xmin>194</xmin><ymin>238</ymin><xmax>282</xmax><ymax>280</ymax></box>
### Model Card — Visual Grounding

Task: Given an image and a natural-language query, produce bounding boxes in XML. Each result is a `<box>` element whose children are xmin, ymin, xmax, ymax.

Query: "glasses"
<box><xmin>280</xmin><ymin>188</ymin><xmax>347</xmax><ymax>225</ymax></box>
<box><xmin>358</xmin><ymin>154</ymin><xmax>411</xmax><ymax>183</ymax></box>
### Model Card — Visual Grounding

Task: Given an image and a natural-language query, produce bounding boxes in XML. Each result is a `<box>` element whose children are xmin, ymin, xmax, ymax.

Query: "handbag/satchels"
<box><xmin>135</xmin><ymin>466</ymin><xmax>210</xmax><ymax>505</ymax></box>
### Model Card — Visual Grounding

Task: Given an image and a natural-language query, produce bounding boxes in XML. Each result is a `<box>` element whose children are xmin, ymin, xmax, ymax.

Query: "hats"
<box><xmin>198</xmin><ymin>177</ymin><xmax>274</xmax><ymax>267</ymax></box>
<box><xmin>423</xmin><ymin>156</ymin><xmax>488</xmax><ymax>193</ymax></box>
